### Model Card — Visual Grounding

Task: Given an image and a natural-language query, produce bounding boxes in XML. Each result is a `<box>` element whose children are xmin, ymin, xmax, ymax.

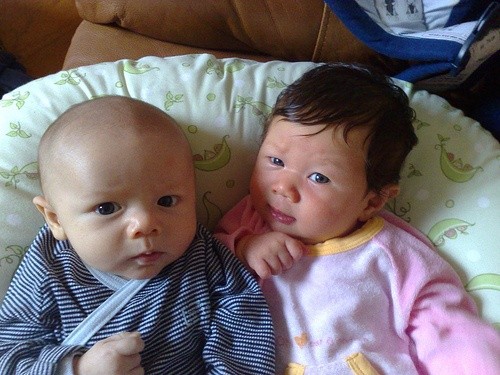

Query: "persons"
<box><xmin>213</xmin><ymin>62</ymin><xmax>500</xmax><ymax>375</ymax></box>
<box><xmin>0</xmin><ymin>95</ymin><xmax>277</xmax><ymax>375</ymax></box>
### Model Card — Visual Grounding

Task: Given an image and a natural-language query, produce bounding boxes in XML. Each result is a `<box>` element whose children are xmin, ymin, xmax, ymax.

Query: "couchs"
<box><xmin>59</xmin><ymin>0</ymin><xmax>500</xmax><ymax>111</ymax></box>
